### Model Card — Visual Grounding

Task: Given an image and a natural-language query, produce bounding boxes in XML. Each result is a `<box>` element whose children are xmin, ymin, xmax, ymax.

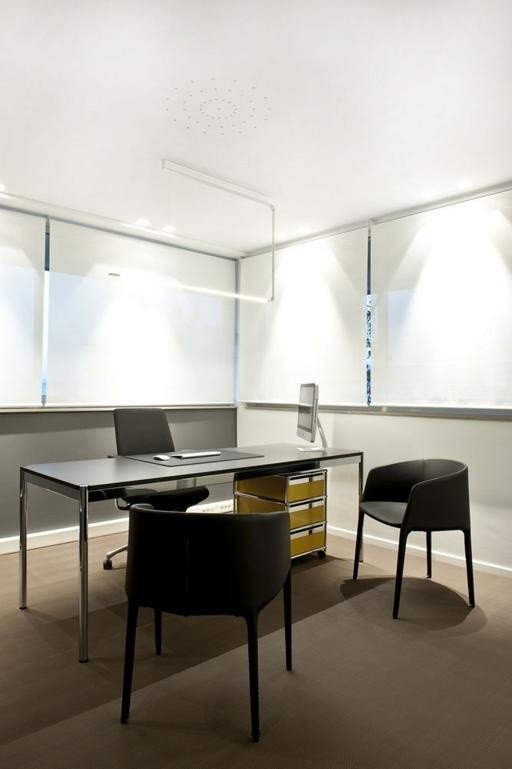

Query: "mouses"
<box><xmin>152</xmin><ymin>454</ymin><xmax>169</xmax><ymax>461</ymax></box>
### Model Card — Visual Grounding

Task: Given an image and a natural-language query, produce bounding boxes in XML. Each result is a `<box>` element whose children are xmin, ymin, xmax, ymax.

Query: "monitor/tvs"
<box><xmin>296</xmin><ymin>383</ymin><xmax>328</xmax><ymax>453</ymax></box>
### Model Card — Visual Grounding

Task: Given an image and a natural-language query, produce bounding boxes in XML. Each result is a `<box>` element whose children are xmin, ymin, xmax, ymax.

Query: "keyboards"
<box><xmin>171</xmin><ymin>451</ymin><xmax>220</xmax><ymax>458</ymax></box>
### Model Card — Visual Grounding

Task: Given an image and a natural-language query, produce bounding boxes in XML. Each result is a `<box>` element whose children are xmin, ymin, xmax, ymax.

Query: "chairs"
<box><xmin>120</xmin><ymin>503</ymin><xmax>293</xmax><ymax>743</ymax></box>
<box><xmin>352</xmin><ymin>457</ymin><xmax>476</xmax><ymax>618</ymax></box>
<box><xmin>101</xmin><ymin>408</ymin><xmax>210</xmax><ymax>569</ymax></box>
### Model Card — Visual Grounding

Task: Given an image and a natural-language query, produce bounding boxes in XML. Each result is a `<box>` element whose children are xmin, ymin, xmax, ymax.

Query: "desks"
<box><xmin>18</xmin><ymin>441</ymin><xmax>364</xmax><ymax>663</ymax></box>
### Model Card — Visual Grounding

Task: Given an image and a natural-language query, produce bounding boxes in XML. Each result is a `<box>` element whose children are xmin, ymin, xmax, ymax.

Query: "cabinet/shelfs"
<box><xmin>233</xmin><ymin>468</ymin><xmax>328</xmax><ymax>560</ymax></box>
